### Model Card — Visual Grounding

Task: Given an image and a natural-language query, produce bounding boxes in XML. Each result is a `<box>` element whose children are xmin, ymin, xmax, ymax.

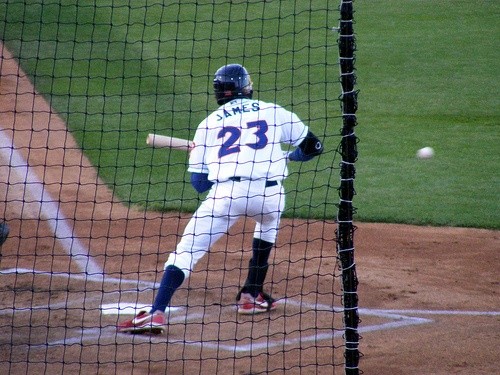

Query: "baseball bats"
<box><xmin>146</xmin><ymin>133</ymin><xmax>291</xmax><ymax>158</ymax></box>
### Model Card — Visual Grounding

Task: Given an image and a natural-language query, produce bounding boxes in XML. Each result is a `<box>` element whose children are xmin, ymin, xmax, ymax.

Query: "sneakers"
<box><xmin>115</xmin><ymin>311</ymin><xmax>165</xmax><ymax>332</ymax></box>
<box><xmin>238</xmin><ymin>292</ymin><xmax>277</xmax><ymax>314</ymax></box>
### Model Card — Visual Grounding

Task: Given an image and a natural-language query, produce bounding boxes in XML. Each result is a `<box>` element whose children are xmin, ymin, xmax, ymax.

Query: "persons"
<box><xmin>116</xmin><ymin>64</ymin><xmax>325</xmax><ymax>335</ymax></box>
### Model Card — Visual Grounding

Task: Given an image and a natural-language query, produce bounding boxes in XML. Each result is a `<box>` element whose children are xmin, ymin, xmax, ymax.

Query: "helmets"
<box><xmin>214</xmin><ymin>64</ymin><xmax>253</xmax><ymax>106</ymax></box>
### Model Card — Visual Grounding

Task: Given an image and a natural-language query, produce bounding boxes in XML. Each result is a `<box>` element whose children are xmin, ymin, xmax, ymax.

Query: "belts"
<box><xmin>216</xmin><ymin>177</ymin><xmax>277</xmax><ymax>187</ymax></box>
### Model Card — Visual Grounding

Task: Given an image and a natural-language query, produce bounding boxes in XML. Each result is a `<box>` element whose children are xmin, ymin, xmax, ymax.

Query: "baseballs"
<box><xmin>417</xmin><ymin>147</ymin><xmax>434</xmax><ymax>159</ymax></box>
